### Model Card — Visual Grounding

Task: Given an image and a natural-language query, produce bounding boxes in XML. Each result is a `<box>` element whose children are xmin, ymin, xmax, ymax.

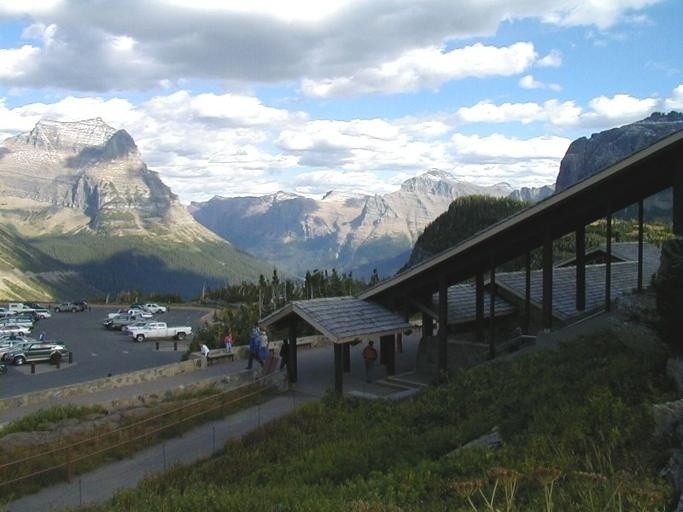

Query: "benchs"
<box><xmin>205</xmin><ymin>352</ymin><xmax>234</xmax><ymax>365</ymax></box>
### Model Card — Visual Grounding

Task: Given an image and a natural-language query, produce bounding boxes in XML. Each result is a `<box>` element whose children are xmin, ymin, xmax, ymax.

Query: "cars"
<box><xmin>55</xmin><ymin>301</ymin><xmax>84</xmax><ymax>313</ymax></box>
<box><xmin>0</xmin><ymin>303</ymin><xmax>69</xmax><ymax>365</ymax></box>
<box><xmin>103</xmin><ymin>301</ymin><xmax>167</xmax><ymax>331</ymax></box>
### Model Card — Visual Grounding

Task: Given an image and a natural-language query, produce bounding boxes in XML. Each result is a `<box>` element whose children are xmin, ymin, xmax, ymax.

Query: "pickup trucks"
<box><xmin>133</xmin><ymin>321</ymin><xmax>193</xmax><ymax>342</ymax></box>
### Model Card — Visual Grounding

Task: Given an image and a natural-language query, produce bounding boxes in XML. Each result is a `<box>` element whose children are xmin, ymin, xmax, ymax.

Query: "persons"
<box><xmin>37</xmin><ymin>332</ymin><xmax>47</xmax><ymax>341</ymax></box>
<box><xmin>224</xmin><ymin>332</ymin><xmax>233</xmax><ymax>353</ymax></box>
<box><xmin>254</xmin><ymin>327</ymin><xmax>269</xmax><ymax>362</ymax></box>
<box><xmin>244</xmin><ymin>327</ymin><xmax>264</xmax><ymax>370</ymax></box>
<box><xmin>199</xmin><ymin>342</ymin><xmax>210</xmax><ymax>360</ymax></box>
<box><xmin>10</xmin><ymin>332</ymin><xmax>16</xmax><ymax>340</ymax></box>
<box><xmin>279</xmin><ymin>337</ymin><xmax>289</xmax><ymax>371</ymax></box>
<box><xmin>361</xmin><ymin>339</ymin><xmax>378</xmax><ymax>382</ymax></box>
